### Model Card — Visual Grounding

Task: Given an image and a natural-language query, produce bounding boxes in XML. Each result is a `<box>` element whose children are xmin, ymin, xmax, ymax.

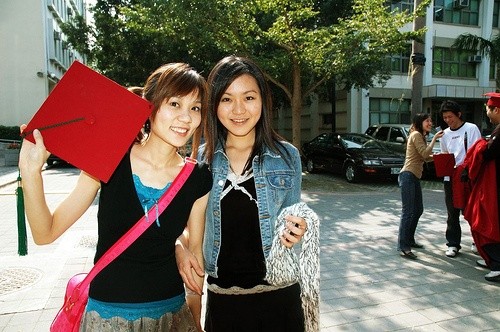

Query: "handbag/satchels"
<box><xmin>49</xmin><ymin>273</ymin><xmax>90</xmax><ymax>332</ymax></box>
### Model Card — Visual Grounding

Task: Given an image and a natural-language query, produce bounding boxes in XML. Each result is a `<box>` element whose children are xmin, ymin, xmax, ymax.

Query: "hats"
<box><xmin>482</xmin><ymin>92</ymin><xmax>500</xmax><ymax>109</ymax></box>
<box><xmin>15</xmin><ymin>61</ymin><xmax>155</xmax><ymax>257</ymax></box>
<box><xmin>432</xmin><ymin>153</ymin><xmax>457</xmax><ymax>177</ymax></box>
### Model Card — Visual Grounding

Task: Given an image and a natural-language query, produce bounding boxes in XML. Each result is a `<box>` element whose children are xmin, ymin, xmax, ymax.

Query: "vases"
<box><xmin>4</xmin><ymin>148</ymin><xmax>20</xmax><ymax>166</ymax></box>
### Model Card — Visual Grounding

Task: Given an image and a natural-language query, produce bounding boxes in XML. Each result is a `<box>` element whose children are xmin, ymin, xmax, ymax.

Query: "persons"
<box><xmin>477</xmin><ymin>92</ymin><xmax>500</xmax><ymax>283</ymax></box>
<box><xmin>397</xmin><ymin>114</ymin><xmax>443</xmax><ymax>260</ymax></box>
<box><xmin>439</xmin><ymin>100</ymin><xmax>482</xmax><ymax>258</ymax></box>
<box><xmin>175</xmin><ymin>54</ymin><xmax>320</xmax><ymax>332</ymax></box>
<box><xmin>17</xmin><ymin>63</ymin><xmax>214</xmax><ymax>332</ymax></box>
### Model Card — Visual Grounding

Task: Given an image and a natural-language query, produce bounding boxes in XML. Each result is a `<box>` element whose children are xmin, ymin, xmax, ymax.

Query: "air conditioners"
<box><xmin>69</xmin><ymin>8</ymin><xmax>72</xmax><ymax>15</ymax></box>
<box><xmin>471</xmin><ymin>55</ymin><xmax>481</xmax><ymax>62</ymax></box>
<box><xmin>457</xmin><ymin>0</ymin><xmax>469</xmax><ymax>7</ymax></box>
<box><xmin>55</xmin><ymin>32</ymin><xmax>61</xmax><ymax>40</ymax></box>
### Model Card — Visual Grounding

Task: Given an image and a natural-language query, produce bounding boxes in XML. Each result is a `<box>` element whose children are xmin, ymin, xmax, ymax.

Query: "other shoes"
<box><xmin>485</xmin><ymin>270</ymin><xmax>500</xmax><ymax>281</ymax></box>
<box><xmin>471</xmin><ymin>243</ymin><xmax>479</xmax><ymax>254</ymax></box>
<box><xmin>445</xmin><ymin>246</ymin><xmax>457</xmax><ymax>257</ymax></box>
<box><xmin>400</xmin><ymin>249</ymin><xmax>417</xmax><ymax>259</ymax></box>
<box><xmin>476</xmin><ymin>259</ymin><xmax>490</xmax><ymax>269</ymax></box>
<box><xmin>412</xmin><ymin>244</ymin><xmax>423</xmax><ymax>248</ymax></box>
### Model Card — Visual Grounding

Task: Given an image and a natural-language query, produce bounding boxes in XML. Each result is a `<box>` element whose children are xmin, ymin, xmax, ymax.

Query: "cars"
<box><xmin>300</xmin><ymin>132</ymin><xmax>405</xmax><ymax>184</ymax></box>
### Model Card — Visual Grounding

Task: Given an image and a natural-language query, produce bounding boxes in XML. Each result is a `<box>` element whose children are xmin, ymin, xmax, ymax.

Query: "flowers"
<box><xmin>8</xmin><ymin>143</ymin><xmax>20</xmax><ymax>149</ymax></box>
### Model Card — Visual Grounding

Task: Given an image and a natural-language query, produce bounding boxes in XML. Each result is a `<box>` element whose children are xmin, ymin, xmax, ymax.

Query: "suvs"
<box><xmin>365</xmin><ymin>124</ymin><xmax>439</xmax><ymax>176</ymax></box>
